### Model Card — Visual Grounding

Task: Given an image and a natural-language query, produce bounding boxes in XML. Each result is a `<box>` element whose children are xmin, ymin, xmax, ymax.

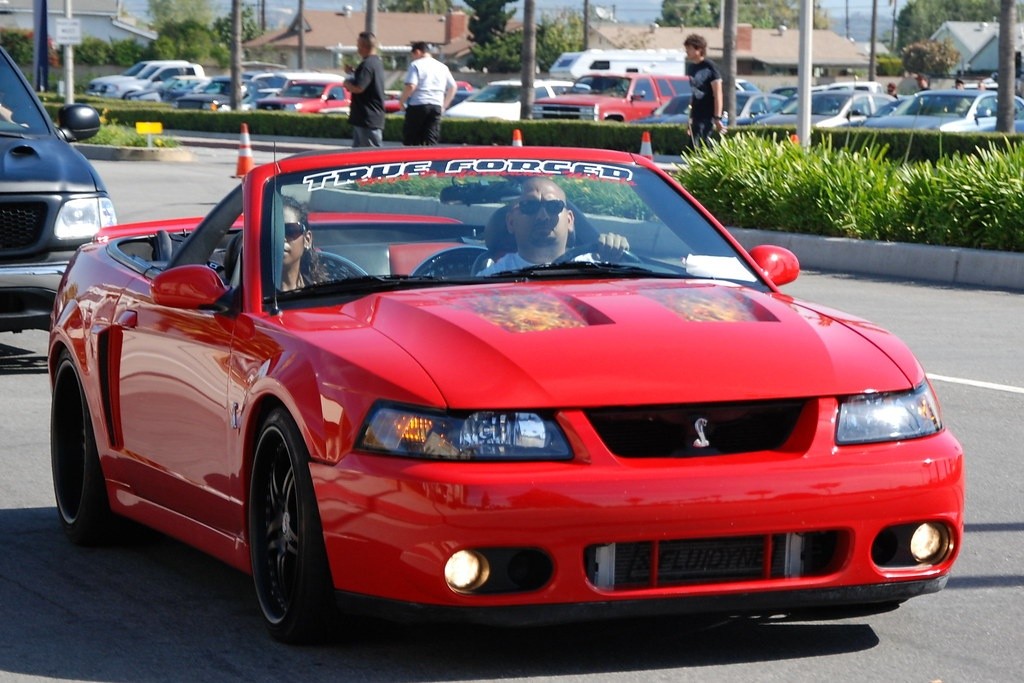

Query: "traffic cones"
<box><xmin>512</xmin><ymin>127</ymin><xmax>524</xmax><ymax>146</ymax></box>
<box><xmin>636</xmin><ymin>131</ymin><xmax>655</xmax><ymax>163</ymax></box>
<box><xmin>231</xmin><ymin>121</ymin><xmax>261</xmax><ymax>180</ymax></box>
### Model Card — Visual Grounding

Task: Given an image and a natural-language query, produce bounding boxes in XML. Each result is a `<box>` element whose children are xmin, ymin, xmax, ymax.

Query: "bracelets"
<box><xmin>713</xmin><ymin>115</ymin><xmax>722</xmax><ymax>119</ymax></box>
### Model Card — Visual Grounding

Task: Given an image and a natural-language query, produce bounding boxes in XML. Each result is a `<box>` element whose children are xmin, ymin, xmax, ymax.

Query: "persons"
<box><xmin>475</xmin><ymin>176</ymin><xmax>629</xmax><ymax>276</ymax></box>
<box><xmin>955</xmin><ymin>80</ymin><xmax>964</xmax><ymax>89</ymax></box>
<box><xmin>887</xmin><ymin>82</ymin><xmax>897</xmax><ymax>97</ymax></box>
<box><xmin>683</xmin><ymin>34</ymin><xmax>723</xmax><ymax>155</ymax></box>
<box><xmin>230</xmin><ymin>195</ymin><xmax>330</xmax><ymax>300</ymax></box>
<box><xmin>977</xmin><ymin>82</ymin><xmax>986</xmax><ymax>90</ymax></box>
<box><xmin>915</xmin><ymin>74</ymin><xmax>930</xmax><ymax>91</ymax></box>
<box><xmin>399</xmin><ymin>41</ymin><xmax>457</xmax><ymax>146</ymax></box>
<box><xmin>343</xmin><ymin>31</ymin><xmax>384</xmax><ymax>147</ymax></box>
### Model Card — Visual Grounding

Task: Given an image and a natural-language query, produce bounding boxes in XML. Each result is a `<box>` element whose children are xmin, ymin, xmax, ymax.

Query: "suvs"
<box><xmin>532</xmin><ymin>71</ymin><xmax>696</xmax><ymax>121</ymax></box>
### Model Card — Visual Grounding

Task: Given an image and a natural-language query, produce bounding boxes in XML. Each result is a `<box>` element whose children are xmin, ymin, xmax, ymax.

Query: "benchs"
<box><xmin>319</xmin><ymin>242</ymin><xmax>486</xmax><ymax>279</ymax></box>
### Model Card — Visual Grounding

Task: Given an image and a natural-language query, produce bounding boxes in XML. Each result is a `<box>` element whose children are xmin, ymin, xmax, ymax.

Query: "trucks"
<box><xmin>547</xmin><ymin>48</ymin><xmax>691</xmax><ymax>81</ymax></box>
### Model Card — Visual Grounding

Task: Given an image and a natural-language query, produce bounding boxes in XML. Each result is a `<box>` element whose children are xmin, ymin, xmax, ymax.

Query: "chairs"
<box><xmin>473</xmin><ymin>199</ymin><xmax>599</xmax><ymax>275</ymax></box>
<box><xmin>232</xmin><ymin>254</ymin><xmax>367</xmax><ymax>291</ymax></box>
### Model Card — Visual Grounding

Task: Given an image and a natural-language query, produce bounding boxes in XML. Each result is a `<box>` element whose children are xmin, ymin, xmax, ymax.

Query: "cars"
<box><xmin>833</xmin><ymin>78</ymin><xmax>1024</xmax><ymax>136</ymax></box>
<box><xmin>750</xmin><ymin>89</ymin><xmax>898</xmax><ymax>129</ymax></box>
<box><xmin>0</xmin><ymin>39</ymin><xmax>119</xmax><ymax>267</ymax></box>
<box><xmin>87</xmin><ymin>60</ymin><xmax>479</xmax><ymax>118</ymax></box>
<box><xmin>630</xmin><ymin>74</ymin><xmax>885</xmax><ymax>127</ymax></box>
<box><xmin>442</xmin><ymin>79</ymin><xmax>592</xmax><ymax>121</ymax></box>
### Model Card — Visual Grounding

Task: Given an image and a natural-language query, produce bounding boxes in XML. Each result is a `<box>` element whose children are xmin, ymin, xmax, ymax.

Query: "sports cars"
<box><xmin>48</xmin><ymin>143</ymin><xmax>966</xmax><ymax>649</ymax></box>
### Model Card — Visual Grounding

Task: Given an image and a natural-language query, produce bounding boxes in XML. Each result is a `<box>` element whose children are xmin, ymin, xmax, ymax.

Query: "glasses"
<box><xmin>519</xmin><ymin>199</ymin><xmax>569</xmax><ymax>214</ymax></box>
<box><xmin>285</xmin><ymin>222</ymin><xmax>306</xmax><ymax>240</ymax></box>
